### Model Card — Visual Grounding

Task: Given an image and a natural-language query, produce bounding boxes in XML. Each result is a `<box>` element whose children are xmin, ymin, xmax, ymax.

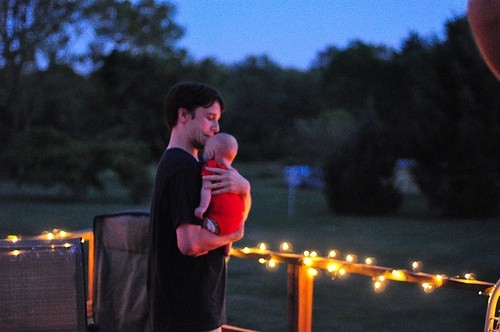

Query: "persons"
<box><xmin>145</xmin><ymin>81</ymin><xmax>253</xmax><ymax>332</ymax></box>
<box><xmin>194</xmin><ymin>133</ymin><xmax>247</xmax><ymax>256</ymax></box>
